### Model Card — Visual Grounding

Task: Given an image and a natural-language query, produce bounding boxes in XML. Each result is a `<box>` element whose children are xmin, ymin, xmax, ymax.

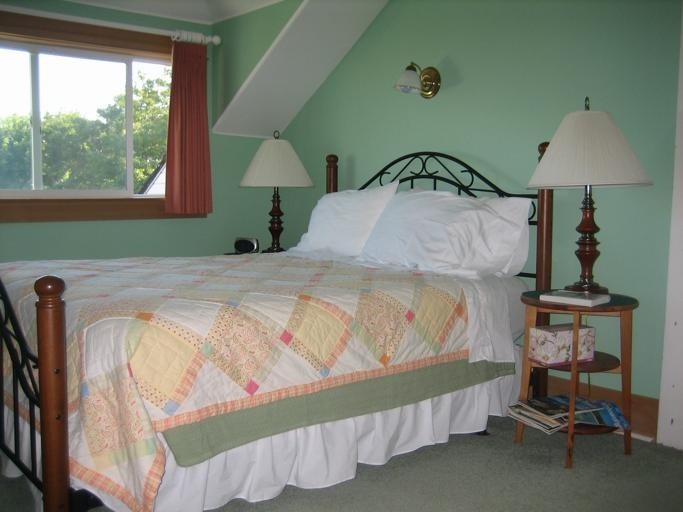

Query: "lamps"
<box><xmin>239</xmin><ymin>130</ymin><xmax>314</xmax><ymax>253</ymax></box>
<box><xmin>393</xmin><ymin>62</ymin><xmax>440</xmax><ymax>98</ymax></box>
<box><xmin>526</xmin><ymin>96</ymin><xmax>654</xmax><ymax>295</ymax></box>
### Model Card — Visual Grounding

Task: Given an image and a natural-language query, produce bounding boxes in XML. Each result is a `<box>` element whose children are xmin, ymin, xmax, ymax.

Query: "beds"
<box><xmin>0</xmin><ymin>142</ymin><xmax>553</xmax><ymax>512</ymax></box>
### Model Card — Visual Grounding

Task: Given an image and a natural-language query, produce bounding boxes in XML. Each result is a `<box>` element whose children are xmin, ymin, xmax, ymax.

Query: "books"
<box><xmin>540</xmin><ymin>289</ymin><xmax>611</xmax><ymax>309</ymax></box>
<box><xmin>505</xmin><ymin>392</ymin><xmax>631</xmax><ymax>436</ymax></box>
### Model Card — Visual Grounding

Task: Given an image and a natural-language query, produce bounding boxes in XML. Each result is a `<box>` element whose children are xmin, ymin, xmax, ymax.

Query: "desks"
<box><xmin>514</xmin><ymin>287</ymin><xmax>639</xmax><ymax>468</ymax></box>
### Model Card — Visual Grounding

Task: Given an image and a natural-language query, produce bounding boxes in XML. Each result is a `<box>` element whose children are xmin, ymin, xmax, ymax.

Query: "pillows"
<box><xmin>285</xmin><ymin>179</ymin><xmax>533</xmax><ymax>282</ymax></box>
